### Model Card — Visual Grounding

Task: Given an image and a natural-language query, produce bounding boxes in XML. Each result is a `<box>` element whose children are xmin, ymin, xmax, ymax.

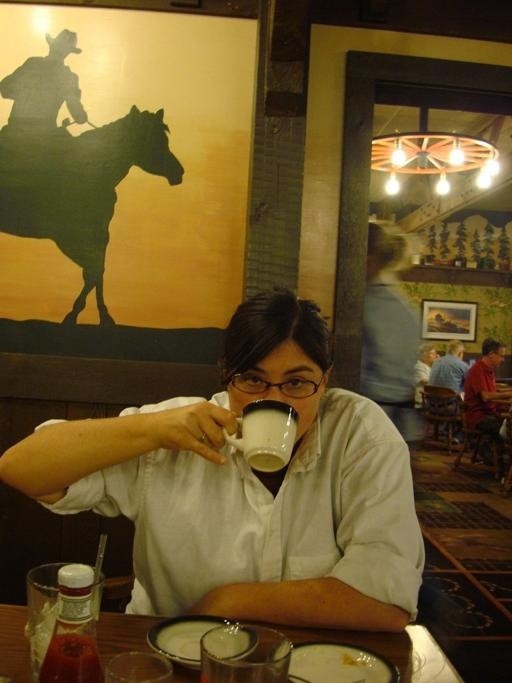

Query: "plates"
<box><xmin>146</xmin><ymin>615</ymin><xmax>267</xmax><ymax>667</ymax></box>
<box><xmin>286</xmin><ymin>643</ymin><xmax>406</xmax><ymax>682</ymax></box>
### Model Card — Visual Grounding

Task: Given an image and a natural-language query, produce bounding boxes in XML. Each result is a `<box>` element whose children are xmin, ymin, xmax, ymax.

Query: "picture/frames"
<box><xmin>421</xmin><ymin>298</ymin><xmax>479</xmax><ymax>345</ymax></box>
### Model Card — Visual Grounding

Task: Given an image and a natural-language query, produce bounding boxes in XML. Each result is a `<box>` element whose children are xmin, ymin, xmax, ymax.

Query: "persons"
<box><xmin>0</xmin><ymin>288</ymin><xmax>425</xmax><ymax>637</ymax></box>
<box><xmin>426</xmin><ymin>340</ymin><xmax>470</xmax><ymax>444</ymax></box>
<box><xmin>360</xmin><ymin>223</ymin><xmax>420</xmax><ymax>440</ymax></box>
<box><xmin>413</xmin><ymin>344</ymin><xmax>440</xmax><ymax>411</ymax></box>
<box><xmin>463</xmin><ymin>337</ymin><xmax>512</xmax><ymax>477</ymax></box>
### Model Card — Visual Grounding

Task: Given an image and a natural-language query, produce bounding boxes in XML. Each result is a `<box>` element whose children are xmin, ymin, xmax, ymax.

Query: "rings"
<box><xmin>199</xmin><ymin>432</ymin><xmax>206</xmax><ymax>441</ymax></box>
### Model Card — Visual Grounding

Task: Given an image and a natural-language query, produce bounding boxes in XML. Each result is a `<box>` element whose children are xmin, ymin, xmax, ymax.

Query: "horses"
<box><xmin>1</xmin><ymin>106</ymin><xmax>186</xmax><ymax>328</ymax></box>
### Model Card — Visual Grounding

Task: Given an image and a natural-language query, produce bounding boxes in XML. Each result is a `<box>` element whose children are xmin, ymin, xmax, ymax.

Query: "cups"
<box><xmin>26</xmin><ymin>564</ymin><xmax>102</xmax><ymax>682</ymax></box>
<box><xmin>196</xmin><ymin>625</ymin><xmax>292</xmax><ymax>682</ymax></box>
<box><xmin>103</xmin><ymin>651</ymin><xmax>175</xmax><ymax>682</ymax></box>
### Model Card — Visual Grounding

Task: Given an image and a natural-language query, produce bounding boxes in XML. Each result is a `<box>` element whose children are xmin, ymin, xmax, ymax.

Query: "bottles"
<box><xmin>219</xmin><ymin>399</ymin><xmax>299</xmax><ymax>473</ymax></box>
<box><xmin>41</xmin><ymin>562</ymin><xmax>102</xmax><ymax>681</ymax></box>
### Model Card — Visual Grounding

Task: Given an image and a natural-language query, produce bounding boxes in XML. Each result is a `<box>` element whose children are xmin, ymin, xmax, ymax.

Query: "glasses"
<box><xmin>231</xmin><ymin>370</ymin><xmax>326</xmax><ymax>399</ymax></box>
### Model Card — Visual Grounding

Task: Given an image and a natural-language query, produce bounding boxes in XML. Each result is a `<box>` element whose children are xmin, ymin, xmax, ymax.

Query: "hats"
<box><xmin>44</xmin><ymin>28</ymin><xmax>84</xmax><ymax>55</ymax></box>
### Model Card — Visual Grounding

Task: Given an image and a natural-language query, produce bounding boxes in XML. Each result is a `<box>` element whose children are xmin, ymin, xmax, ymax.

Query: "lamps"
<box><xmin>370</xmin><ymin>98</ymin><xmax>501</xmax><ymax>196</ymax></box>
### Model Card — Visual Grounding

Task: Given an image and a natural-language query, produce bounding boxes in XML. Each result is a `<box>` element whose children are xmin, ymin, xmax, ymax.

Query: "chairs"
<box><xmin>408</xmin><ymin>374</ymin><xmax>510</xmax><ymax>485</ymax></box>
